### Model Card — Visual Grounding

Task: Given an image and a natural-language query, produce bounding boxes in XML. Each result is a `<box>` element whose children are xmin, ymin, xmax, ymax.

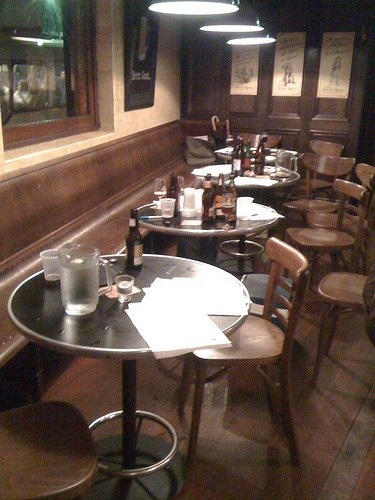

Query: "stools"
<box><xmin>0</xmin><ymin>132</ymin><xmax>374</xmax><ymax>500</ymax></box>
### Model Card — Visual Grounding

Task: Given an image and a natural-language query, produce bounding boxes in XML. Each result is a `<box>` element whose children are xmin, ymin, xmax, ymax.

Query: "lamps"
<box><xmin>148</xmin><ymin>0</ymin><xmax>276</xmax><ymax>45</ymax></box>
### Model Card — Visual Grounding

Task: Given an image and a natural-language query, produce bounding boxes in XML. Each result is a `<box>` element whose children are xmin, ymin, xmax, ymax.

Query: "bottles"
<box><xmin>215</xmin><ymin>173</ymin><xmax>226</xmax><ymax>219</ymax></box>
<box><xmin>228</xmin><ymin>174</ymin><xmax>238</xmax><ymax>223</ymax></box>
<box><xmin>126</xmin><ymin>208</ymin><xmax>143</xmax><ymax>271</ymax></box>
<box><xmin>232</xmin><ymin>135</ymin><xmax>244</xmax><ymax>177</ymax></box>
<box><xmin>254</xmin><ymin>139</ymin><xmax>266</xmax><ymax>175</ymax></box>
<box><xmin>242</xmin><ymin>140</ymin><xmax>252</xmax><ymax>172</ymax></box>
<box><xmin>204</xmin><ymin>171</ymin><xmax>214</xmax><ymax>223</ymax></box>
<box><xmin>166</xmin><ymin>171</ymin><xmax>180</xmax><ymax>213</ymax></box>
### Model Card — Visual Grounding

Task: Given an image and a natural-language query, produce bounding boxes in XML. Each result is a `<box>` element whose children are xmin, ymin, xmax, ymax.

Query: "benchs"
<box><xmin>178</xmin><ymin>119</ymin><xmax>348</xmax><ymax>163</ymax></box>
<box><xmin>0</xmin><ymin>120</ymin><xmax>207</xmax><ymax>371</ymax></box>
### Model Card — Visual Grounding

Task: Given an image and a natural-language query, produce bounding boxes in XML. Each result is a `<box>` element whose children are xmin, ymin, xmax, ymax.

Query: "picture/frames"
<box><xmin>125</xmin><ymin>0</ymin><xmax>159</xmax><ymax>112</ymax></box>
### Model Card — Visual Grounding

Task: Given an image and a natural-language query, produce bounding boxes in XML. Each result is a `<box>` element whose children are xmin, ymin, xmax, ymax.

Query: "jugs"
<box><xmin>58</xmin><ymin>241</ymin><xmax>112</xmax><ymax>316</ymax></box>
<box><xmin>276</xmin><ymin>149</ymin><xmax>297</xmax><ymax>178</ymax></box>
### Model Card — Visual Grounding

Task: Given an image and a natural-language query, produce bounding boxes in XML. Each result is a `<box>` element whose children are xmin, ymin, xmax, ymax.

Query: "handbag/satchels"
<box><xmin>184</xmin><ymin>134</ymin><xmax>217</xmax><ymax>166</ymax></box>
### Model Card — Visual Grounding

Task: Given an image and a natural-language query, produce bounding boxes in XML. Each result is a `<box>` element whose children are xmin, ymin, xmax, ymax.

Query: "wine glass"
<box><xmin>154</xmin><ymin>177</ymin><xmax>167</xmax><ymax>209</ymax></box>
<box><xmin>221</xmin><ymin>192</ymin><xmax>236</xmax><ymax>230</ymax></box>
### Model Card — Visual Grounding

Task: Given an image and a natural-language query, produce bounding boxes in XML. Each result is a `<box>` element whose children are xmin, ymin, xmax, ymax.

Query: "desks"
<box><xmin>136</xmin><ymin>202</ymin><xmax>278</xmax><ymax>265</ymax></box>
<box><xmin>6</xmin><ymin>254</ymin><xmax>250</xmax><ymax>500</ymax></box>
<box><xmin>195</xmin><ymin>165</ymin><xmax>301</xmax><ymax>273</ymax></box>
<box><xmin>217</xmin><ymin>148</ymin><xmax>302</xmax><ymax>166</ymax></box>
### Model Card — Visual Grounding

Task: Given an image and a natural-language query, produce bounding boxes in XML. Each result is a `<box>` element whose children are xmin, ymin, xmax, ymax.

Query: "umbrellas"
<box><xmin>211</xmin><ymin>115</ymin><xmax>225</xmax><ymax>165</ymax></box>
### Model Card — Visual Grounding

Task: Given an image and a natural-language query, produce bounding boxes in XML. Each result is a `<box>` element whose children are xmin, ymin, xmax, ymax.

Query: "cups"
<box><xmin>39</xmin><ymin>249</ymin><xmax>60</xmax><ymax>282</ymax></box>
<box><xmin>114</xmin><ymin>275</ymin><xmax>135</xmax><ymax>304</ymax></box>
<box><xmin>160</xmin><ymin>198</ymin><xmax>177</xmax><ymax>219</ymax></box>
<box><xmin>177</xmin><ymin>177</ymin><xmax>197</xmax><ymax>211</ymax></box>
<box><xmin>238</xmin><ymin>196</ymin><xmax>255</xmax><ymax>221</ymax></box>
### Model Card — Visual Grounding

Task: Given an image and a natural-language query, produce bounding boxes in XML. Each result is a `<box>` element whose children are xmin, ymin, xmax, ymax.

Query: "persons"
<box><xmin>0</xmin><ymin>63</ymin><xmax>66</xmax><ymax>112</ymax></box>
